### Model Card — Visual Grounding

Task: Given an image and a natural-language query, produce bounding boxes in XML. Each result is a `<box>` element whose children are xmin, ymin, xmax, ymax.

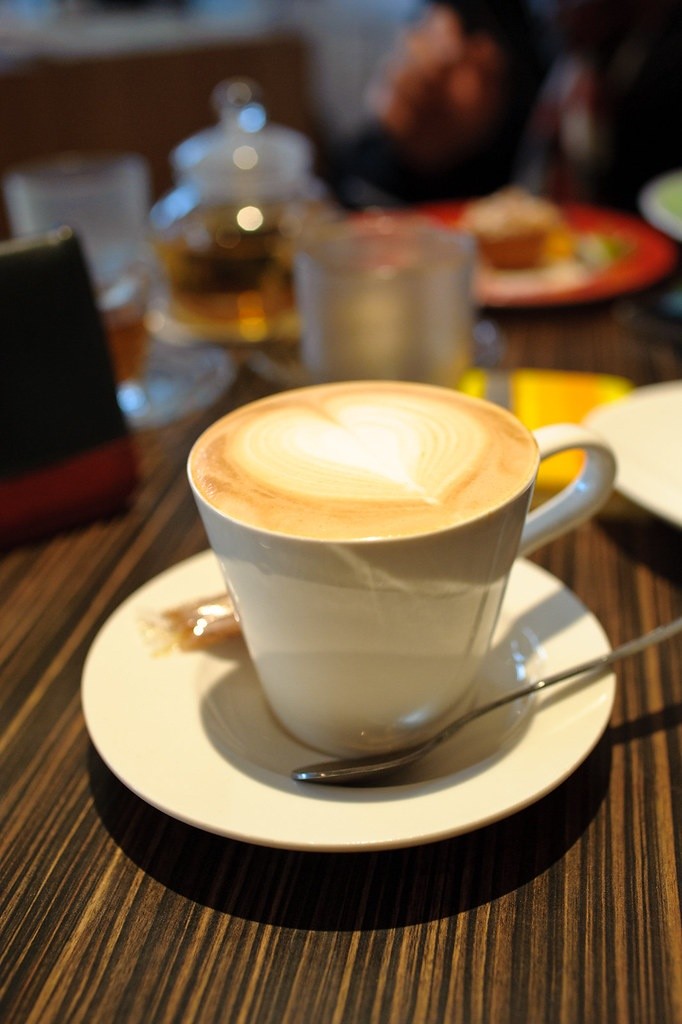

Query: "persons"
<box><xmin>324</xmin><ymin>0</ymin><xmax>547</xmax><ymax>213</ymax></box>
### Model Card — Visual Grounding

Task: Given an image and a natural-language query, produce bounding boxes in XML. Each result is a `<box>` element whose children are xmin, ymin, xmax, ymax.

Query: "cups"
<box><xmin>294</xmin><ymin>222</ymin><xmax>476</xmax><ymax>390</ymax></box>
<box><xmin>3</xmin><ymin>157</ymin><xmax>150</xmax><ymax>416</ymax></box>
<box><xmin>0</xmin><ymin>230</ymin><xmax>135</xmax><ymax>544</ymax></box>
<box><xmin>184</xmin><ymin>378</ymin><xmax>618</xmax><ymax>757</ymax></box>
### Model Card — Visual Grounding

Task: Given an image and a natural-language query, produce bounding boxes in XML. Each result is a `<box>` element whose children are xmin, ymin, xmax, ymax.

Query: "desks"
<box><xmin>0</xmin><ymin>291</ymin><xmax>682</xmax><ymax>1024</ymax></box>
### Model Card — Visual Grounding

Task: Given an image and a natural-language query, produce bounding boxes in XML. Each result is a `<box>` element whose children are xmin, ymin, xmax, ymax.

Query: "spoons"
<box><xmin>291</xmin><ymin>617</ymin><xmax>682</xmax><ymax>781</ymax></box>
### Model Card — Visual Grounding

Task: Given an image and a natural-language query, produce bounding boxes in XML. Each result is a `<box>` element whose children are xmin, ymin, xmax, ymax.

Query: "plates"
<box><xmin>584</xmin><ymin>378</ymin><xmax>682</xmax><ymax>527</ymax></box>
<box><xmin>347</xmin><ymin>204</ymin><xmax>674</xmax><ymax>306</ymax></box>
<box><xmin>637</xmin><ymin>173</ymin><xmax>682</xmax><ymax>239</ymax></box>
<box><xmin>80</xmin><ymin>546</ymin><xmax>615</xmax><ymax>852</ymax></box>
